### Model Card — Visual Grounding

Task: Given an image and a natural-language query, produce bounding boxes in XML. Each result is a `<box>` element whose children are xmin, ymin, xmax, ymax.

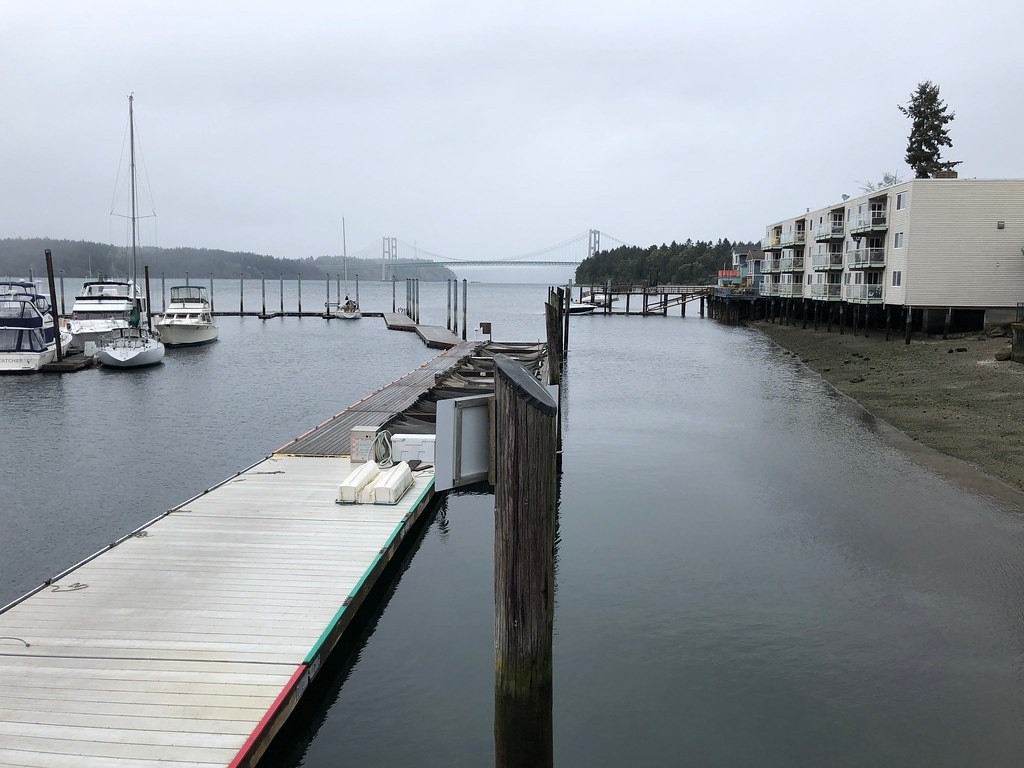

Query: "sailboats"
<box><xmin>336</xmin><ymin>216</ymin><xmax>365</xmax><ymax>321</ymax></box>
<box><xmin>89</xmin><ymin>91</ymin><xmax>168</xmax><ymax>370</ymax></box>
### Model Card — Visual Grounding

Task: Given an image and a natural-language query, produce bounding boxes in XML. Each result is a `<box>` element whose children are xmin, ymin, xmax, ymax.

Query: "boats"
<box><xmin>0</xmin><ymin>276</ymin><xmax>74</xmax><ymax>373</ymax></box>
<box><xmin>62</xmin><ymin>269</ymin><xmax>152</xmax><ymax>353</ymax></box>
<box><xmin>153</xmin><ymin>285</ymin><xmax>224</xmax><ymax>348</ymax></box>
<box><xmin>561</xmin><ymin>297</ymin><xmax>598</xmax><ymax>316</ymax></box>
<box><xmin>584</xmin><ymin>294</ymin><xmax>620</xmax><ymax>307</ymax></box>
